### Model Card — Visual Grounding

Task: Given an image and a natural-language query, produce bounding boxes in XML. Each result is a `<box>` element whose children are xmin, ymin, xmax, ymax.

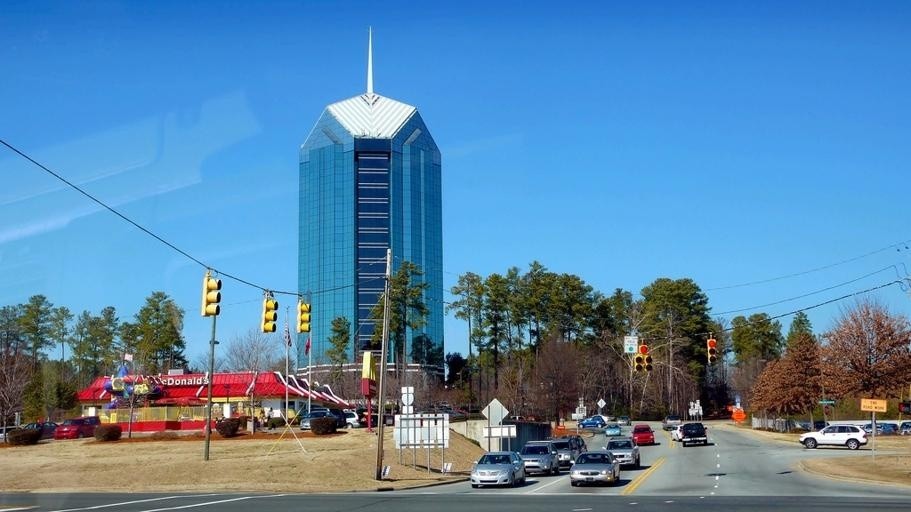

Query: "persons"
<box><xmin>265</xmin><ymin>406</ymin><xmax>274</xmax><ymax>430</ymax></box>
<box><xmin>258</xmin><ymin>409</ymin><xmax>266</xmax><ymax>431</ymax></box>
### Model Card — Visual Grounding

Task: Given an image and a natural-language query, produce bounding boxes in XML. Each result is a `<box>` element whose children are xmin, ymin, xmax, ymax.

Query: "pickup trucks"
<box><xmin>682</xmin><ymin>423</ymin><xmax>707</xmax><ymax>446</ymax></box>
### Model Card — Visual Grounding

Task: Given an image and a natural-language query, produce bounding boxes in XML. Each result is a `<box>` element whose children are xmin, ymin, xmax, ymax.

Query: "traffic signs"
<box><xmin>861</xmin><ymin>398</ymin><xmax>887</xmax><ymax>412</ymax></box>
<box><xmin>818</xmin><ymin>400</ymin><xmax>834</xmax><ymax>405</ymax></box>
<box><xmin>623</xmin><ymin>336</ymin><xmax>637</xmax><ymax>353</ymax></box>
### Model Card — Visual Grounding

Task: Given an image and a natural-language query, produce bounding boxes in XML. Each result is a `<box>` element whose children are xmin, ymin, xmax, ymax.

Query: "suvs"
<box><xmin>799</xmin><ymin>425</ymin><xmax>868</xmax><ymax>450</ymax></box>
<box><xmin>520</xmin><ymin>441</ymin><xmax>560</xmax><ymax>478</ymax></box>
<box><xmin>601</xmin><ymin>437</ymin><xmax>640</xmax><ymax>469</ymax></box>
<box><xmin>53</xmin><ymin>417</ymin><xmax>101</xmax><ymax>438</ymax></box>
<box><xmin>549</xmin><ymin>438</ymin><xmax>579</xmax><ymax>468</ymax></box>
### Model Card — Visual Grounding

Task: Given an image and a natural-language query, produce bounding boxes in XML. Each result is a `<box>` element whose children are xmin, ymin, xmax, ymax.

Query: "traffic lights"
<box><xmin>707</xmin><ymin>339</ymin><xmax>716</xmax><ymax>363</ymax></box>
<box><xmin>260</xmin><ymin>290</ymin><xmax>279</xmax><ymax>333</ymax></box>
<box><xmin>644</xmin><ymin>355</ymin><xmax>652</xmax><ymax>371</ymax></box>
<box><xmin>298</xmin><ymin>300</ymin><xmax>311</xmax><ymax>333</ymax></box>
<box><xmin>638</xmin><ymin>345</ymin><xmax>647</xmax><ymax>354</ymax></box>
<box><xmin>202</xmin><ymin>272</ymin><xmax>222</xmax><ymax>317</ymax></box>
<box><xmin>899</xmin><ymin>403</ymin><xmax>910</xmax><ymax>412</ymax></box>
<box><xmin>634</xmin><ymin>355</ymin><xmax>642</xmax><ymax>371</ymax></box>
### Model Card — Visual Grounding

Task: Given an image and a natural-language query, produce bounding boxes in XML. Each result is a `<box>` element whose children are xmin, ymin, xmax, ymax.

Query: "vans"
<box><xmin>661</xmin><ymin>415</ymin><xmax>680</xmax><ymax>430</ymax></box>
<box><xmin>342</xmin><ymin>412</ymin><xmax>366</xmax><ymax>429</ymax></box>
<box><xmin>301</xmin><ymin>408</ymin><xmax>343</xmax><ymax>428</ymax></box>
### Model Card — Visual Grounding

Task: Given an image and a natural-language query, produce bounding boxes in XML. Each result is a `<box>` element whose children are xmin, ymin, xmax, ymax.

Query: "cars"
<box><xmin>569</xmin><ymin>451</ymin><xmax>620</xmax><ymax>488</ymax></box>
<box><xmin>864</xmin><ymin>422</ymin><xmax>911</xmax><ymax>435</ymax></box>
<box><xmin>361</xmin><ymin>415</ymin><xmax>377</xmax><ymax>427</ymax></box>
<box><xmin>616</xmin><ymin>416</ymin><xmax>631</xmax><ymax>426</ymax></box>
<box><xmin>605</xmin><ymin>424</ymin><xmax>621</xmax><ymax>437</ymax></box>
<box><xmin>0</xmin><ymin>427</ymin><xmax>21</xmax><ymax>443</ymax></box>
<box><xmin>468</xmin><ymin>451</ymin><xmax>525</xmax><ymax>488</ymax></box>
<box><xmin>562</xmin><ymin>435</ymin><xmax>587</xmax><ymax>453</ymax></box>
<box><xmin>670</xmin><ymin>425</ymin><xmax>682</xmax><ymax>442</ymax></box>
<box><xmin>416</xmin><ymin>401</ymin><xmax>468</xmax><ymax>417</ymax></box>
<box><xmin>576</xmin><ymin>415</ymin><xmax>606</xmax><ymax>429</ymax></box>
<box><xmin>632</xmin><ymin>425</ymin><xmax>654</xmax><ymax>444</ymax></box>
<box><xmin>299</xmin><ymin>411</ymin><xmax>339</xmax><ymax>430</ymax></box>
<box><xmin>510</xmin><ymin>416</ymin><xmax>525</xmax><ymax>421</ymax></box>
<box><xmin>23</xmin><ymin>422</ymin><xmax>58</xmax><ymax>439</ymax></box>
<box><xmin>526</xmin><ymin>417</ymin><xmax>545</xmax><ymax>422</ymax></box>
